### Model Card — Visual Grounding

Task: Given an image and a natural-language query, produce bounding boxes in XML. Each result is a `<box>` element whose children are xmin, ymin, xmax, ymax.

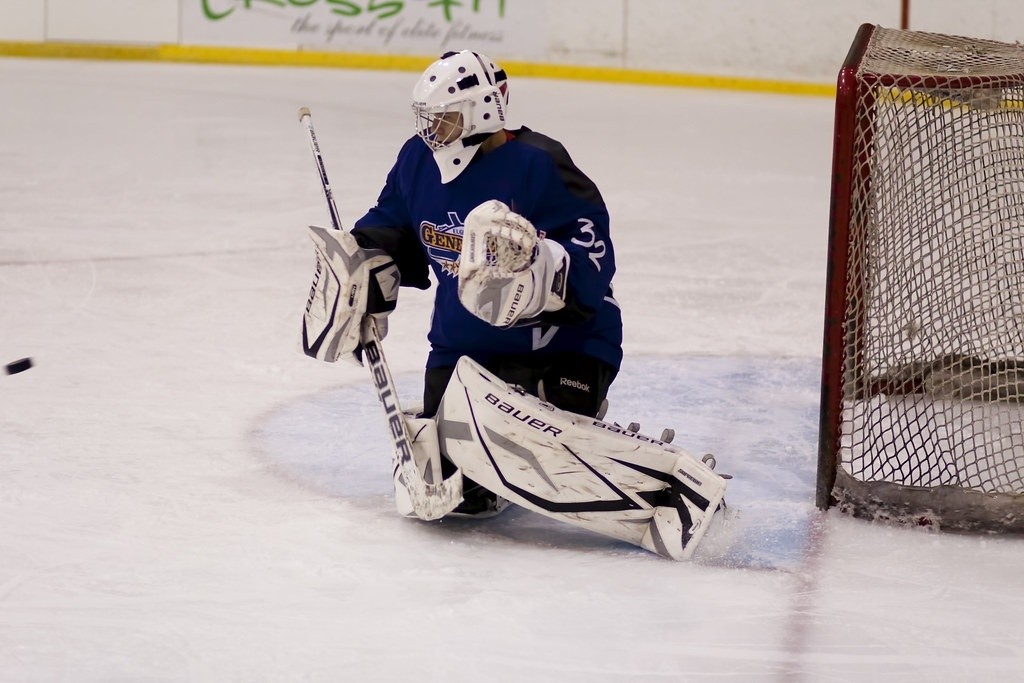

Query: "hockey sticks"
<box><xmin>298</xmin><ymin>106</ymin><xmax>467</xmax><ymax>521</ymax></box>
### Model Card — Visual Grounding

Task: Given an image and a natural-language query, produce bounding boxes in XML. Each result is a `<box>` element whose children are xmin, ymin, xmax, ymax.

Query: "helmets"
<box><xmin>412</xmin><ymin>50</ymin><xmax>510</xmax><ymax>184</ymax></box>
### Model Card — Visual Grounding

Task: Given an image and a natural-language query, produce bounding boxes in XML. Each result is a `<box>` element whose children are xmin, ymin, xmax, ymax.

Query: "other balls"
<box><xmin>5</xmin><ymin>358</ymin><xmax>33</xmax><ymax>375</ymax></box>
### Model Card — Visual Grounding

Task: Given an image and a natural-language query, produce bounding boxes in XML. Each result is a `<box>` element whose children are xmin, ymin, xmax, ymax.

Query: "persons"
<box><xmin>303</xmin><ymin>48</ymin><xmax>624</xmax><ymax>520</ymax></box>
